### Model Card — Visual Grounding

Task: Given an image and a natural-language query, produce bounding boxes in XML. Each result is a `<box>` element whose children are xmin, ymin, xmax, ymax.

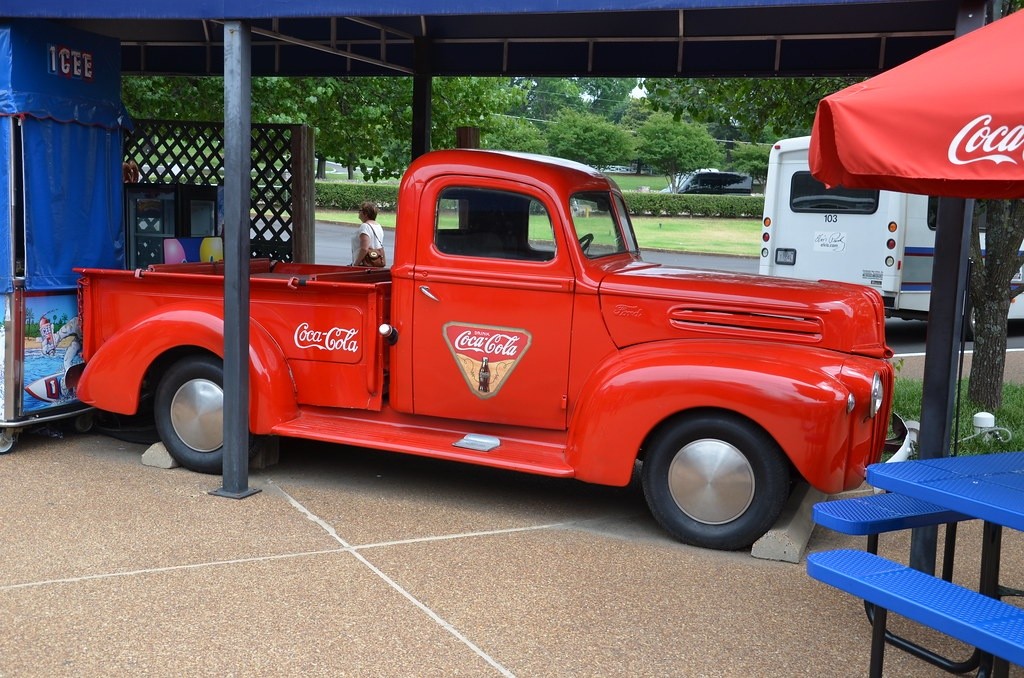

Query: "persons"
<box><xmin>349</xmin><ymin>202</ymin><xmax>387</xmax><ymax>267</ymax></box>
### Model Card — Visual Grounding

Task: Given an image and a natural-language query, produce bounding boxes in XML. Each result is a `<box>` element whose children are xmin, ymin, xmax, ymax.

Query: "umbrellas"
<box><xmin>808</xmin><ymin>8</ymin><xmax>1023</xmax><ymax>198</ymax></box>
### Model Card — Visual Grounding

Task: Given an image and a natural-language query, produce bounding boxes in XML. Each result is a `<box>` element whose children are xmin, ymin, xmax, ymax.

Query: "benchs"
<box><xmin>806</xmin><ymin>493</ymin><xmax>1022</xmax><ymax>678</ymax></box>
<box><xmin>436</xmin><ymin>226</ymin><xmax>537</xmax><ymax>262</ymax></box>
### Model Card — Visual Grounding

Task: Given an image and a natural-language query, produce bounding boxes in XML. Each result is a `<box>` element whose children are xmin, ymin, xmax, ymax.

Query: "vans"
<box><xmin>657</xmin><ymin>167</ymin><xmax>753</xmax><ymax>198</ymax></box>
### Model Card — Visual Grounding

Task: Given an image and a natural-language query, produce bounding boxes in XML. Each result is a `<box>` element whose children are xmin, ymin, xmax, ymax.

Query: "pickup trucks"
<box><xmin>71</xmin><ymin>148</ymin><xmax>895</xmax><ymax>553</ymax></box>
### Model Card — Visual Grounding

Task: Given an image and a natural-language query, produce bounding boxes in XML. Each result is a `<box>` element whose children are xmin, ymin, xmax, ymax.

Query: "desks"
<box><xmin>866</xmin><ymin>448</ymin><xmax>1023</xmax><ymax>678</ymax></box>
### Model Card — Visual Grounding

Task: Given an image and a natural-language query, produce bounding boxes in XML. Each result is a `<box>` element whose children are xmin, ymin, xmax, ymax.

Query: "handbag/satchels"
<box><xmin>362</xmin><ymin>223</ymin><xmax>386</xmax><ymax>267</ymax></box>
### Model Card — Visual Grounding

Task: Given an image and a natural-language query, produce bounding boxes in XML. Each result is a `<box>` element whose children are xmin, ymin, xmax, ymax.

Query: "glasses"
<box><xmin>357</xmin><ymin>209</ymin><xmax>364</xmax><ymax>213</ymax></box>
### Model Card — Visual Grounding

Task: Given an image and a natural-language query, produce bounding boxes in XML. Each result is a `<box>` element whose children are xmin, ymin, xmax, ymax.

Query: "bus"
<box><xmin>759</xmin><ymin>136</ymin><xmax>1024</xmax><ymax>342</ymax></box>
